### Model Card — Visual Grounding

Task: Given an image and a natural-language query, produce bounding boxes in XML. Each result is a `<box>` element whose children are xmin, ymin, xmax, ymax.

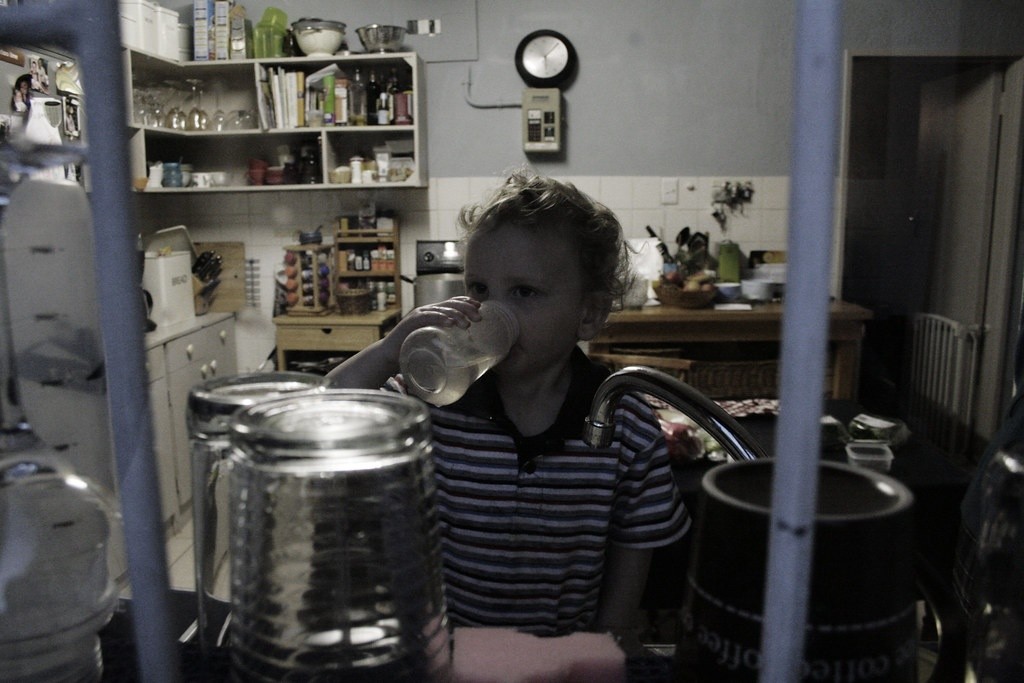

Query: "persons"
<box><xmin>11</xmin><ymin>72</ymin><xmax>30</xmax><ymax>112</ymax></box>
<box><xmin>67</xmin><ymin>104</ymin><xmax>76</xmax><ymax>133</ymax></box>
<box><xmin>31</xmin><ymin>57</ymin><xmax>47</xmax><ymax>90</ymax></box>
<box><xmin>321</xmin><ymin>163</ymin><xmax>693</xmax><ymax>647</ymax></box>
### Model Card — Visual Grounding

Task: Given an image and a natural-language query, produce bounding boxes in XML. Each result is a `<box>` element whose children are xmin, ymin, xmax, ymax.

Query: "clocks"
<box><xmin>515</xmin><ymin>29</ymin><xmax>576</xmax><ymax>87</ymax></box>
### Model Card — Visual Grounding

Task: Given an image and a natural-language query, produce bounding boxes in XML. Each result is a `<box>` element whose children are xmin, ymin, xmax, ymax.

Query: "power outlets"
<box><xmin>660</xmin><ymin>178</ymin><xmax>679</xmax><ymax>206</ymax></box>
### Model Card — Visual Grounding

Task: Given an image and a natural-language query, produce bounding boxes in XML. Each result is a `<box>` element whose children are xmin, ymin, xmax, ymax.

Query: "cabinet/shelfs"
<box><xmin>181</xmin><ymin>50</ymin><xmax>429</xmax><ymax>193</ymax></box>
<box><xmin>120</xmin><ymin>48</ymin><xmax>182</xmax><ymax>193</ymax></box>
<box><xmin>17</xmin><ymin>311</ymin><xmax>239</xmax><ymax>526</ymax></box>
<box><xmin>333</xmin><ymin>217</ymin><xmax>401</xmax><ymax>308</ymax></box>
<box><xmin>283</xmin><ymin>242</ymin><xmax>335</xmax><ymax>316</ymax></box>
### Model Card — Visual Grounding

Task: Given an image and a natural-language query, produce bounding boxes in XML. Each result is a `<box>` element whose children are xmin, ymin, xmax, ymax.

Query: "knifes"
<box><xmin>192</xmin><ymin>250</ymin><xmax>226</xmax><ymax>299</ymax></box>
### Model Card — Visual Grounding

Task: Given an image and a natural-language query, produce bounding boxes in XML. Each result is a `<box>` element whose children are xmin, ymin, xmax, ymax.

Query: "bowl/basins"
<box><xmin>356</xmin><ymin>24</ymin><xmax>408</xmax><ymax>53</ymax></box>
<box><xmin>291</xmin><ymin>17</ymin><xmax>347</xmax><ymax>57</ymax></box>
<box><xmin>714</xmin><ymin>279</ymin><xmax>779</xmax><ymax>301</ymax></box>
<box><xmin>653</xmin><ymin>288</ymin><xmax>718</xmax><ymax>309</ymax></box>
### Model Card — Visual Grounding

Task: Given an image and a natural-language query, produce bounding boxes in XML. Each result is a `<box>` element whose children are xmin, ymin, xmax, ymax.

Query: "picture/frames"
<box><xmin>60</xmin><ymin>97</ymin><xmax>81</xmax><ymax>137</ymax></box>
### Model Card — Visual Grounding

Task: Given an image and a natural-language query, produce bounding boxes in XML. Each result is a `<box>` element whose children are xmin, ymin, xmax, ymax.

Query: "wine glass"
<box><xmin>134</xmin><ymin>79</ymin><xmax>257</xmax><ymax>132</ymax></box>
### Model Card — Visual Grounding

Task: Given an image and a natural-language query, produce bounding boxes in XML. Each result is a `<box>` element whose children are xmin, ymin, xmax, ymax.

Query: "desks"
<box><xmin>737</xmin><ymin>401</ymin><xmax>974</xmax><ymax>604</ymax></box>
<box><xmin>271</xmin><ymin>309</ymin><xmax>402</xmax><ymax>371</ymax></box>
<box><xmin>588</xmin><ymin>299</ymin><xmax>873</xmax><ymax>400</ymax></box>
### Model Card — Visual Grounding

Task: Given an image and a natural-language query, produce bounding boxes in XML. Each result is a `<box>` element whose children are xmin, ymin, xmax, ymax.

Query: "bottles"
<box><xmin>349</xmin><ymin>68</ymin><xmax>412</xmax><ymax>125</ymax></box>
<box><xmin>187</xmin><ymin>370</ymin><xmax>453</xmax><ymax>680</ymax></box>
<box><xmin>656</xmin><ymin>242</ymin><xmax>684</xmax><ymax>289</ymax></box>
<box><xmin>300</xmin><ymin>150</ymin><xmax>322</xmax><ymax>184</ymax></box>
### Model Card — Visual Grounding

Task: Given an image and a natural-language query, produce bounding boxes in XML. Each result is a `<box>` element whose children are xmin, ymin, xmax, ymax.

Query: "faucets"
<box><xmin>581</xmin><ymin>365</ymin><xmax>770</xmax><ymax>463</ymax></box>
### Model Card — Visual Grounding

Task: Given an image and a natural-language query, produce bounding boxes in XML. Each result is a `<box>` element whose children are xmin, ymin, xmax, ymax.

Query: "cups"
<box><xmin>244</xmin><ymin>158</ymin><xmax>284</xmax><ymax>186</ymax></box>
<box><xmin>299</xmin><ymin>232</ymin><xmax>322</xmax><ymax>246</ymax></box>
<box><xmin>401</xmin><ymin>302</ymin><xmax>519</xmax><ymax>407</ymax></box>
<box><xmin>161</xmin><ymin>163</ymin><xmax>232</xmax><ymax>187</ymax></box>
<box><xmin>624</xmin><ymin>278</ymin><xmax>649</xmax><ymax>310</ymax></box>
<box><xmin>673</xmin><ymin>460</ymin><xmax>968</xmax><ymax>680</ymax></box>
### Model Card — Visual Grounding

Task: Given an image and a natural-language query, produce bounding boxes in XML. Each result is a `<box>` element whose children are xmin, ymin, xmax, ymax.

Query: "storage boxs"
<box><xmin>384</xmin><ymin>139</ymin><xmax>414</xmax><ymax>154</ymax></box>
<box><xmin>252</xmin><ymin>24</ymin><xmax>289</xmax><ymax>59</ymax></box>
<box><xmin>120</xmin><ymin>12</ymin><xmax>138</xmax><ymax>47</ymax></box>
<box><xmin>141</xmin><ymin>251</ymin><xmax>195</xmax><ymax>327</ymax></box>
<box><xmin>334</xmin><ymin>87</ymin><xmax>347</xmax><ymax>123</ymax></box>
<box><xmin>119</xmin><ymin>0</ymin><xmax>158</xmax><ymax>54</ymax></box>
<box><xmin>157</xmin><ymin>7</ymin><xmax>180</xmax><ymax>62</ymax></box>
<box><xmin>192</xmin><ymin>0</ymin><xmax>216</xmax><ymax>61</ymax></box>
<box><xmin>390</xmin><ymin>157</ymin><xmax>415</xmax><ymax>182</ymax></box>
<box><xmin>230</xmin><ymin>18</ymin><xmax>254</xmax><ymax>60</ymax></box>
<box><xmin>215</xmin><ymin>1</ymin><xmax>235</xmax><ymax>60</ymax></box>
<box><xmin>333</xmin><ymin>289</ymin><xmax>373</xmax><ymax>317</ymax></box>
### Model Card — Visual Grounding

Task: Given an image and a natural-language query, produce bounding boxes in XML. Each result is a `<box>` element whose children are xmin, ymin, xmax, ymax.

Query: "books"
<box><xmin>254</xmin><ymin>63</ymin><xmax>304</xmax><ymax>131</ymax></box>
